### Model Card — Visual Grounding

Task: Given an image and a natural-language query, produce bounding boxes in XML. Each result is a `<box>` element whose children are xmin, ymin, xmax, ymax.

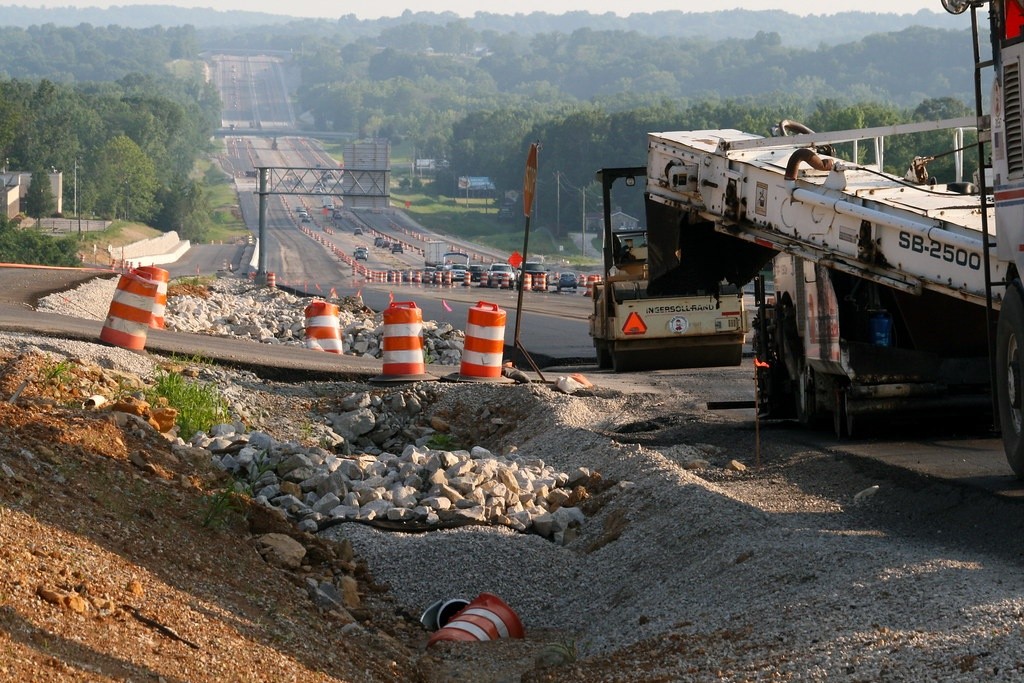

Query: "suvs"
<box><xmin>515</xmin><ymin>262</ymin><xmax>549</xmax><ymax>290</ymax></box>
<box><xmin>487</xmin><ymin>263</ymin><xmax>515</xmax><ymax>290</ymax></box>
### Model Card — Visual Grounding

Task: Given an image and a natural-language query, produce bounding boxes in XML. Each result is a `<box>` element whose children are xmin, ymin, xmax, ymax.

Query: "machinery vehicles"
<box><xmin>645</xmin><ymin>0</ymin><xmax>1024</xmax><ymax>483</ymax></box>
<box><xmin>586</xmin><ymin>166</ymin><xmax>749</xmax><ymax>371</ymax></box>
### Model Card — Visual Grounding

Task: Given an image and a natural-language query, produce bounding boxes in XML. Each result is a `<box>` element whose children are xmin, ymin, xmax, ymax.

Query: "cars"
<box><xmin>422</xmin><ymin>267</ymin><xmax>435</xmax><ymax>282</ymax></box>
<box><xmin>449</xmin><ymin>263</ymin><xmax>469</xmax><ymax>282</ymax></box>
<box><xmin>278</xmin><ymin>170</ymin><xmax>404</xmax><ymax>261</ymax></box>
<box><xmin>434</xmin><ymin>266</ymin><xmax>450</xmax><ymax>282</ymax></box>
<box><xmin>469</xmin><ymin>265</ymin><xmax>486</xmax><ymax>282</ymax></box>
<box><xmin>557</xmin><ymin>272</ymin><xmax>577</xmax><ymax>291</ymax></box>
